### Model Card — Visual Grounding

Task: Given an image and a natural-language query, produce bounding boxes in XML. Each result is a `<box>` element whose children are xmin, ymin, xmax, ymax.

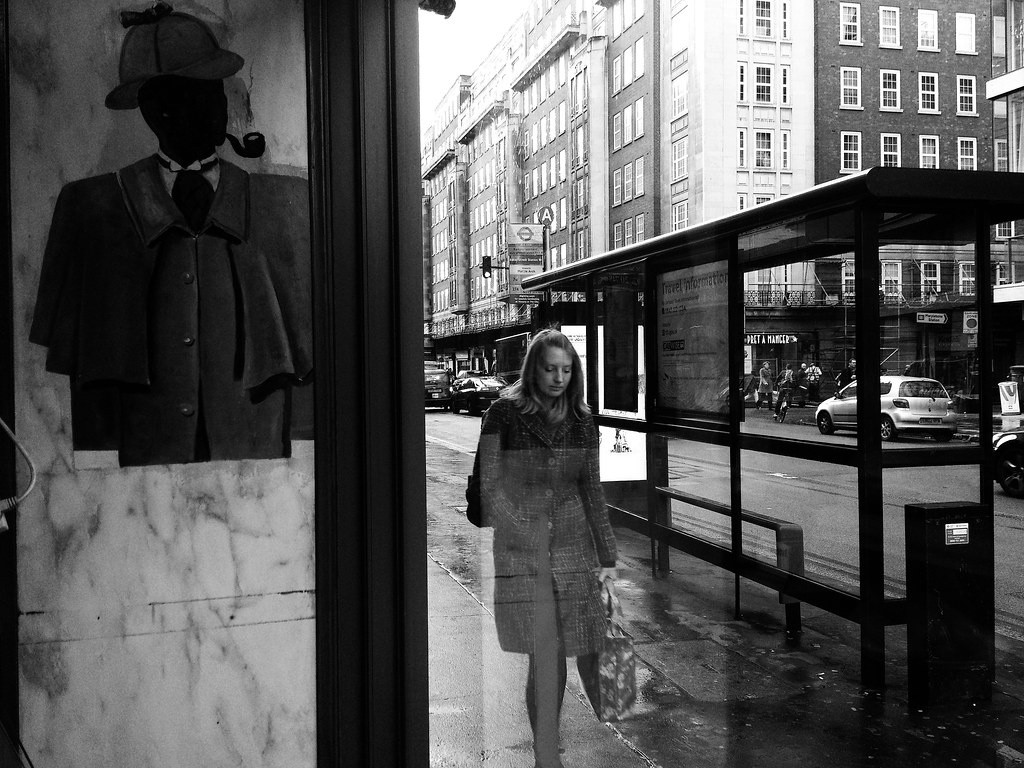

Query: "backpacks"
<box><xmin>784</xmin><ymin>370</ymin><xmax>793</xmax><ymax>383</ymax></box>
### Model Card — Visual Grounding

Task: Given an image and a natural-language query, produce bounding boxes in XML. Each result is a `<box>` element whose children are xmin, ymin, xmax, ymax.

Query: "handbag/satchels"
<box><xmin>466</xmin><ymin>397</ymin><xmax>516</xmax><ymax>527</ymax></box>
<box><xmin>577</xmin><ymin>574</ymin><xmax>636</xmax><ymax>723</ymax></box>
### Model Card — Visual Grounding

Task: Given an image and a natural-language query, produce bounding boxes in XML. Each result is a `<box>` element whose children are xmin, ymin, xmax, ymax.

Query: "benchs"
<box><xmin>655</xmin><ymin>487</ymin><xmax>805</xmax><ymax>630</ymax></box>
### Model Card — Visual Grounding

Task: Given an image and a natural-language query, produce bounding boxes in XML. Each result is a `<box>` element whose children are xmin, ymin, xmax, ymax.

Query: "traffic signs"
<box><xmin>916</xmin><ymin>312</ymin><xmax>948</xmax><ymax>324</ymax></box>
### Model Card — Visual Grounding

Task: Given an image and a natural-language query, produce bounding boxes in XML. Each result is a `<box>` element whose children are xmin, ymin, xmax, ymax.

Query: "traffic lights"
<box><xmin>482</xmin><ymin>255</ymin><xmax>491</xmax><ymax>279</ymax></box>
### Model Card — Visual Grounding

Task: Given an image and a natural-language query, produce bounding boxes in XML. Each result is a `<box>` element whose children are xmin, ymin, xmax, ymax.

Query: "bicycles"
<box><xmin>777</xmin><ymin>388</ymin><xmax>792</xmax><ymax>424</ymax></box>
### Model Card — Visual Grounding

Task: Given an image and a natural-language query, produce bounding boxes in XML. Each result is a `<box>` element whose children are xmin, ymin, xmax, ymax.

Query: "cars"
<box><xmin>814</xmin><ymin>376</ymin><xmax>958</xmax><ymax>442</ymax></box>
<box><xmin>451</xmin><ymin>376</ymin><xmax>506</xmax><ymax>417</ymax></box>
<box><xmin>992</xmin><ymin>431</ymin><xmax>1024</xmax><ymax>499</ymax></box>
<box><xmin>423</xmin><ymin>360</ymin><xmax>509</xmax><ymax>412</ymax></box>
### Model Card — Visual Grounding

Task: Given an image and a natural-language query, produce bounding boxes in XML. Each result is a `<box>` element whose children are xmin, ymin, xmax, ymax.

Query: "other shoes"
<box><xmin>773</xmin><ymin>414</ymin><xmax>777</xmax><ymax>417</ymax></box>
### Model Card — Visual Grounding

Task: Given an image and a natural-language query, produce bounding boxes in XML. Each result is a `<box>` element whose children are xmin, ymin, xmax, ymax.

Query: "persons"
<box><xmin>838</xmin><ymin>358</ymin><xmax>856</xmax><ymax>390</ymax></box>
<box><xmin>756</xmin><ymin>361</ymin><xmax>774</xmax><ymax>411</ymax></box>
<box><xmin>880</xmin><ymin>366</ymin><xmax>887</xmax><ymax>375</ymax></box>
<box><xmin>797</xmin><ymin>362</ymin><xmax>808</xmax><ymax>407</ymax></box>
<box><xmin>447</xmin><ymin>368</ymin><xmax>457</xmax><ymax>384</ymax></box>
<box><xmin>772</xmin><ymin>363</ymin><xmax>796</xmax><ymax>418</ymax></box>
<box><xmin>474</xmin><ymin>329</ymin><xmax>619</xmax><ymax>768</ymax></box>
<box><xmin>804</xmin><ymin>363</ymin><xmax>822</xmax><ymax>402</ymax></box>
